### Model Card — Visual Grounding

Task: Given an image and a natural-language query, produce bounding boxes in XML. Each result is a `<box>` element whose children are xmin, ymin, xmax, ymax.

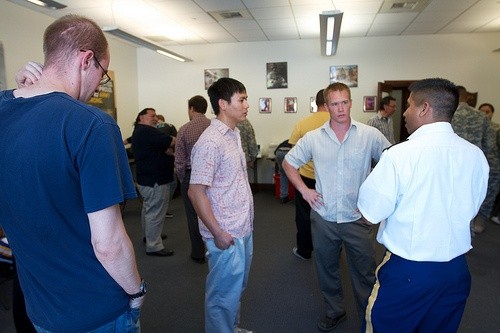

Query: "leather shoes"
<box><xmin>145</xmin><ymin>250</ymin><xmax>174</xmax><ymax>257</ymax></box>
<box><xmin>143</xmin><ymin>234</ymin><xmax>167</xmax><ymax>242</ymax></box>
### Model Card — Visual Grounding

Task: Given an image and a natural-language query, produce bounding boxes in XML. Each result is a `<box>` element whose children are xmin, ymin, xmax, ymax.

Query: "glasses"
<box><xmin>80</xmin><ymin>48</ymin><xmax>111</xmax><ymax>86</ymax></box>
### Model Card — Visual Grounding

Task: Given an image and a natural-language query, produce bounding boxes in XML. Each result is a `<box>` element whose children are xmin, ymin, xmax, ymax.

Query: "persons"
<box><xmin>267</xmin><ymin>63</ymin><xmax>285</xmax><ymax>88</ymax></box>
<box><xmin>237</xmin><ymin>118</ymin><xmax>259</xmax><ymax>174</ymax></box>
<box><xmin>356</xmin><ymin>78</ymin><xmax>490</xmax><ymax>333</ymax></box>
<box><xmin>287</xmin><ymin>89</ymin><xmax>331</xmax><ymax>260</ymax></box>
<box><xmin>263</xmin><ymin>99</ymin><xmax>270</xmax><ymax>112</ymax></box>
<box><xmin>0</xmin><ymin>14</ymin><xmax>147</xmax><ymax>333</ymax></box>
<box><xmin>124</xmin><ymin>108</ymin><xmax>177</xmax><ymax>257</ymax></box>
<box><xmin>368</xmin><ymin>96</ymin><xmax>397</xmax><ymax>169</ymax></box>
<box><xmin>187</xmin><ymin>78</ymin><xmax>254</xmax><ymax>333</ymax></box>
<box><xmin>451</xmin><ymin>86</ymin><xmax>500</xmax><ymax>237</ymax></box>
<box><xmin>175</xmin><ymin>95</ymin><xmax>211</xmax><ymax>264</ymax></box>
<box><xmin>282</xmin><ymin>82</ymin><xmax>393</xmax><ymax>331</ymax></box>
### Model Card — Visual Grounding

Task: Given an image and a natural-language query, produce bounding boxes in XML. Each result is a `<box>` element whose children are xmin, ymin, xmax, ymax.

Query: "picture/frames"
<box><xmin>205</xmin><ymin>68</ymin><xmax>229</xmax><ymax>90</ymax></box>
<box><xmin>284</xmin><ymin>96</ymin><xmax>297</xmax><ymax>113</ymax></box>
<box><xmin>363</xmin><ymin>96</ymin><xmax>377</xmax><ymax>112</ymax></box>
<box><xmin>329</xmin><ymin>64</ymin><xmax>358</xmax><ymax>87</ymax></box>
<box><xmin>259</xmin><ymin>97</ymin><xmax>272</xmax><ymax>113</ymax></box>
<box><xmin>310</xmin><ymin>97</ymin><xmax>317</xmax><ymax>112</ymax></box>
<box><xmin>266</xmin><ymin>62</ymin><xmax>288</xmax><ymax>89</ymax></box>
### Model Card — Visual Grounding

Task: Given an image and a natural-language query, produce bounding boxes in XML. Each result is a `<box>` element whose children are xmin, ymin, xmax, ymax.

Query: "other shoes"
<box><xmin>492</xmin><ymin>217</ymin><xmax>499</xmax><ymax>224</ymax></box>
<box><xmin>318</xmin><ymin>311</ymin><xmax>348</xmax><ymax>330</ymax></box>
<box><xmin>292</xmin><ymin>247</ymin><xmax>308</xmax><ymax>261</ymax></box>
<box><xmin>473</xmin><ymin>223</ymin><xmax>483</xmax><ymax>233</ymax></box>
<box><xmin>190</xmin><ymin>252</ymin><xmax>204</xmax><ymax>264</ymax></box>
<box><xmin>280</xmin><ymin>197</ymin><xmax>289</xmax><ymax>204</ymax></box>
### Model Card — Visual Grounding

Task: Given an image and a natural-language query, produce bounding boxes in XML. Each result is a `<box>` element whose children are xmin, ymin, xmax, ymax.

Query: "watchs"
<box><xmin>127</xmin><ymin>278</ymin><xmax>146</xmax><ymax>299</ymax></box>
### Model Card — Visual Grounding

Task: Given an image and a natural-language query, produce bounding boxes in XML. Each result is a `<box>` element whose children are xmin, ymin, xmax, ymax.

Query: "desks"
<box><xmin>265</xmin><ymin>152</ymin><xmax>278</xmax><ymax>177</ymax></box>
<box><xmin>249</xmin><ymin>153</ymin><xmax>262</xmax><ymax>192</ymax></box>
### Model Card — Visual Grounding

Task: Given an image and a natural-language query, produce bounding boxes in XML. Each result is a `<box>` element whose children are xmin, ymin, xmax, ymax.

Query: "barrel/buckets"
<box><xmin>274</xmin><ymin>173</ymin><xmax>295</xmax><ymax>199</ymax></box>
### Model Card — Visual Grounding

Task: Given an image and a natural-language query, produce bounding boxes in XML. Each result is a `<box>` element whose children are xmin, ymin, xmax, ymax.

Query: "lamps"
<box><xmin>102</xmin><ymin>0</ymin><xmax>193</xmax><ymax>65</ymax></box>
<box><xmin>318</xmin><ymin>0</ymin><xmax>343</xmax><ymax>57</ymax></box>
